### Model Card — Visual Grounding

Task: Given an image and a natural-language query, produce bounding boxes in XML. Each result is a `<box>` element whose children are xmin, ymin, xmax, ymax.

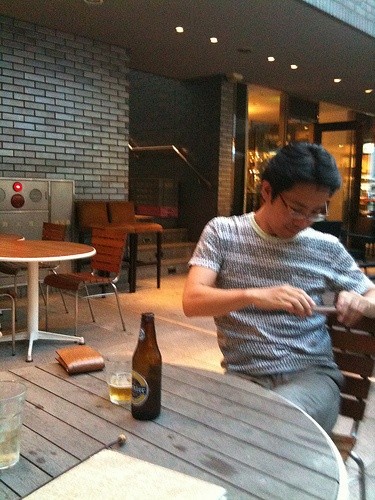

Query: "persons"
<box><xmin>182</xmin><ymin>140</ymin><xmax>375</xmax><ymax>437</ymax></box>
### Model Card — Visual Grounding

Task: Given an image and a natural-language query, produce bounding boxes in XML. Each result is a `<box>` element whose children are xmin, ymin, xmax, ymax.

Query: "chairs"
<box><xmin>0</xmin><ymin>288</ymin><xmax>19</xmax><ymax>356</ymax></box>
<box><xmin>0</xmin><ymin>222</ymin><xmax>68</xmax><ymax>322</ymax></box>
<box><xmin>315</xmin><ymin>220</ymin><xmax>375</xmax><ymax>500</ymax></box>
<box><xmin>43</xmin><ymin>228</ymin><xmax>129</xmax><ymax>343</ymax></box>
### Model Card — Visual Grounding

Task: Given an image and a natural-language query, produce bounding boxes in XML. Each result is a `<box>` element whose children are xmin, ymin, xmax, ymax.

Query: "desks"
<box><xmin>0</xmin><ymin>233</ymin><xmax>96</xmax><ymax>363</ymax></box>
<box><xmin>0</xmin><ymin>360</ymin><xmax>347</xmax><ymax>500</ymax></box>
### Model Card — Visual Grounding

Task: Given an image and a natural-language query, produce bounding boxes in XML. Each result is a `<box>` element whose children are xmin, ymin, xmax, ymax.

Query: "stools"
<box><xmin>74</xmin><ymin>200</ymin><xmax>163</xmax><ymax>298</ymax></box>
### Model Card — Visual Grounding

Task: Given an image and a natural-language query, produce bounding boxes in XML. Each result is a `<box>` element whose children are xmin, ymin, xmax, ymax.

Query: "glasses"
<box><xmin>277</xmin><ymin>191</ymin><xmax>329</xmax><ymax>222</ymax></box>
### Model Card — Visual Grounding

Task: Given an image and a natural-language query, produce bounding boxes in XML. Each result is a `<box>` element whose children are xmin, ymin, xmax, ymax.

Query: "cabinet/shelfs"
<box><xmin>128</xmin><ymin>178</ymin><xmax>178</xmax><ymax>229</ymax></box>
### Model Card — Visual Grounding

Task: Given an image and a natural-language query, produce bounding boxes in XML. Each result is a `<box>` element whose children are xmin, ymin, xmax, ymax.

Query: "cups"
<box><xmin>0</xmin><ymin>380</ymin><xmax>27</xmax><ymax>470</ymax></box>
<box><xmin>107</xmin><ymin>372</ymin><xmax>131</xmax><ymax>405</ymax></box>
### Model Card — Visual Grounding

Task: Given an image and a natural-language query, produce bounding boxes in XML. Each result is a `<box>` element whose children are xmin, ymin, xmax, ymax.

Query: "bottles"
<box><xmin>130</xmin><ymin>312</ymin><xmax>162</xmax><ymax>421</ymax></box>
